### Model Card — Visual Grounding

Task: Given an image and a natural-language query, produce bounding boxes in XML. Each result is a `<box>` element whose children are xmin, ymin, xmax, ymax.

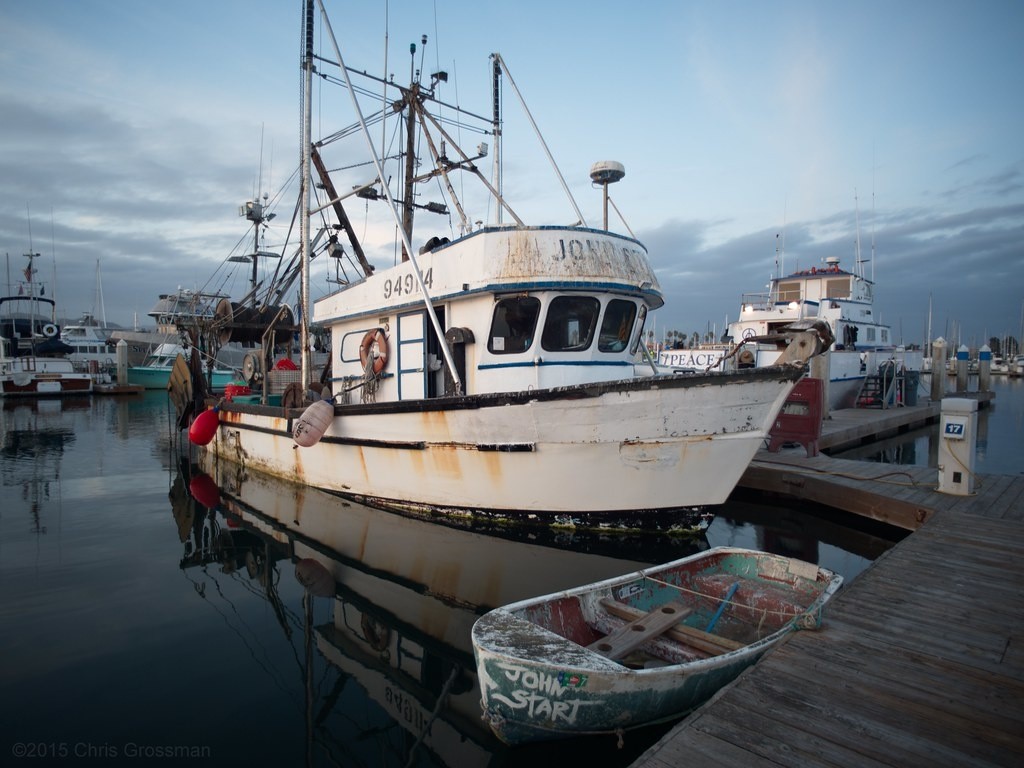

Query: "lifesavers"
<box><xmin>359</xmin><ymin>329</ymin><xmax>389</xmax><ymax>374</ymax></box>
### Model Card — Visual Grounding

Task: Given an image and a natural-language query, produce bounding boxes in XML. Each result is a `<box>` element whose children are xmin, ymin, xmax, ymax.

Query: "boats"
<box><xmin>58</xmin><ymin>120</ymin><xmax>332</xmax><ymax>391</ymax></box>
<box><xmin>470</xmin><ymin>544</ymin><xmax>842</xmax><ymax>748</ymax></box>
<box><xmin>171</xmin><ymin>444</ymin><xmax>713</xmax><ymax>768</ymax></box>
<box><xmin>170</xmin><ymin>1</ymin><xmax>810</xmax><ymax>540</ymax></box>
<box><xmin>655</xmin><ymin>144</ymin><xmax>925</xmax><ymax>413</ymax></box>
<box><xmin>0</xmin><ymin>200</ymin><xmax>93</xmax><ymax>396</ymax></box>
<box><xmin>896</xmin><ymin>292</ymin><xmax>1024</xmax><ymax>378</ymax></box>
